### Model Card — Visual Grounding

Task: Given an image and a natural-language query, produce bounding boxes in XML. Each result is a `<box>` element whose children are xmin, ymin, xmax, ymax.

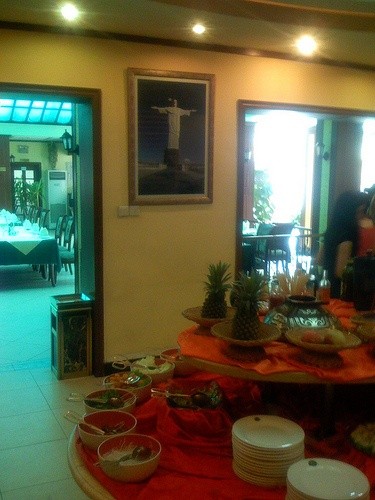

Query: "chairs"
<box><xmin>242</xmin><ymin>221</ymin><xmax>296</xmax><ymax>284</ymax></box>
<box><xmin>28</xmin><ymin>205</ymin><xmax>76</xmax><ymax>283</ymax></box>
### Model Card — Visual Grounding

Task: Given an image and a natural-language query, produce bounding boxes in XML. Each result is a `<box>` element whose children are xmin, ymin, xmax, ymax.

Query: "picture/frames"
<box><xmin>127</xmin><ymin>67</ymin><xmax>216</xmax><ymax>206</ymax></box>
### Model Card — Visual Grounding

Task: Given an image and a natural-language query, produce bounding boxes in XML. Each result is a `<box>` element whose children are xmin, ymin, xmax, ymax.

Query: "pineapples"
<box><xmin>201</xmin><ymin>261</ymin><xmax>231</xmax><ymax>318</ymax></box>
<box><xmin>232</xmin><ymin>268</ymin><xmax>265</xmax><ymax>341</ymax></box>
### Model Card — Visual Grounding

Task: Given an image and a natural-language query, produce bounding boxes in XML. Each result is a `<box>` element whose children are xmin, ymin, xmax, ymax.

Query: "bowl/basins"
<box><xmin>83</xmin><ymin>389</ymin><xmax>136</xmax><ymax>414</ymax></box>
<box><xmin>159</xmin><ymin>348</ymin><xmax>196</xmax><ymax>377</ymax></box>
<box><xmin>97</xmin><ymin>434</ymin><xmax>162</xmax><ymax>482</ymax></box>
<box><xmin>165</xmin><ymin>396</ymin><xmax>244</xmax><ymax>435</ymax></box>
<box><xmin>102</xmin><ymin>372</ymin><xmax>152</xmax><ymax>403</ymax></box>
<box><xmin>77</xmin><ymin>410</ymin><xmax>137</xmax><ymax>451</ymax></box>
<box><xmin>130</xmin><ymin>357</ymin><xmax>175</xmax><ymax>384</ymax></box>
<box><xmin>180</xmin><ymin>305</ymin><xmax>361</xmax><ymax>357</ymax></box>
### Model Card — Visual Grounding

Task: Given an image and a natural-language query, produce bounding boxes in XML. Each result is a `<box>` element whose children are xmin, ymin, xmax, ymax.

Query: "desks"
<box><xmin>242</xmin><ymin>233</ymin><xmax>256</xmax><ymax>271</ymax></box>
<box><xmin>67</xmin><ymin>299</ymin><xmax>375</xmax><ymax>500</ymax></box>
<box><xmin>0</xmin><ymin>226</ymin><xmax>56</xmax><ymax>285</ymax></box>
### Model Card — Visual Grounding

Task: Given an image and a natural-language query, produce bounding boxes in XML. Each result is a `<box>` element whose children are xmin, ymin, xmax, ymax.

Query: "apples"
<box><xmin>300</xmin><ymin>325</ymin><xmax>346</xmax><ymax>344</ymax></box>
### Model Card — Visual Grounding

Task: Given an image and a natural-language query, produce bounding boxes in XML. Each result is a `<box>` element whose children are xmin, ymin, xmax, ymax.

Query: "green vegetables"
<box><xmin>85</xmin><ymin>389</ymin><xmax>123</xmax><ymax>409</ymax></box>
<box><xmin>127</xmin><ymin>366</ymin><xmax>155</xmax><ymax>388</ymax></box>
<box><xmin>207</xmin><ymin>389</ymin><xmax>220</xmax><ymax>404</ymax></box>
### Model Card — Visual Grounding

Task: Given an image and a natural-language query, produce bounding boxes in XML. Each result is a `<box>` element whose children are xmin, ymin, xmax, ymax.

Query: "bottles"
<box><xmin>0</xmin><ymin>208</ymin><xmax>49</xmax><ymax>239</ymax></box>
<box><xmin>317</xmin><ymin>269</ymin><xmax>331</xmax><ymax>305</ymax></box>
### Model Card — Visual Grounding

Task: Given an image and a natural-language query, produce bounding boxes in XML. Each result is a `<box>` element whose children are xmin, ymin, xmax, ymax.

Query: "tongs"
<box><xmin>63</xmin><ymin>410</ymin><xmax>110</xmax><ymax>435</ymax></box>
<box><xmin>112</xmin><ymin>355</ymin><xmax>156</xmax><ymax>370</ymax></box>
<box><xmin>97</xmin><ymin>376</ymin><xmax>140</xmax><ymax>385</ymax></box>
<box><xmin>150</xmin><ymin>388</ymin><xmax>211</xmax><ymax>407</ymax></box>
<box><xmin>66</xmin><ymin>392</ymin><xmax>122</xmax><ymax>407</ymax></box>
<box><xmin>93</xmin><ymin>446</ymin><xmax>150</xmax><ymax>471</ymax></box>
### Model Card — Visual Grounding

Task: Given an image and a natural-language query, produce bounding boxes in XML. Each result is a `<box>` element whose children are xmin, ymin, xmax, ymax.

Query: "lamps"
<box><xmin>10</xmin><ymin>154</ymin><xmax>15</xmax><ymax>167</ymax></box>
<box><xmin>60</xmin><ymin>128</ymin><xmax>80</xmax><ymax>155</ymax></box>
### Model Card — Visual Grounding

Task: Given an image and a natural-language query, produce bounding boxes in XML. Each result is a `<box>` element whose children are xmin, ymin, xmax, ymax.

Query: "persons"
<box><xmin>316</xmin><ymin>190</ymin><xmax>365</xmax><ymax>302</ymax></box>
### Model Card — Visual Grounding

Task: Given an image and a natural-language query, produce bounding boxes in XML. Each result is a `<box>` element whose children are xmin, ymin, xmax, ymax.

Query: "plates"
<box><xmin>231</xmin><ymin>414</ymin><xmax>370</xmax><ymax>500</ymax></box>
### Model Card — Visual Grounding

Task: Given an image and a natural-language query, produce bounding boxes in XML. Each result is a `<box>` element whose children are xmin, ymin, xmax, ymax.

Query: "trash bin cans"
<box><xmin>50</xmin><ymin>292</ymin><xmax>93</xmax><ymax>380</ymax></box>
